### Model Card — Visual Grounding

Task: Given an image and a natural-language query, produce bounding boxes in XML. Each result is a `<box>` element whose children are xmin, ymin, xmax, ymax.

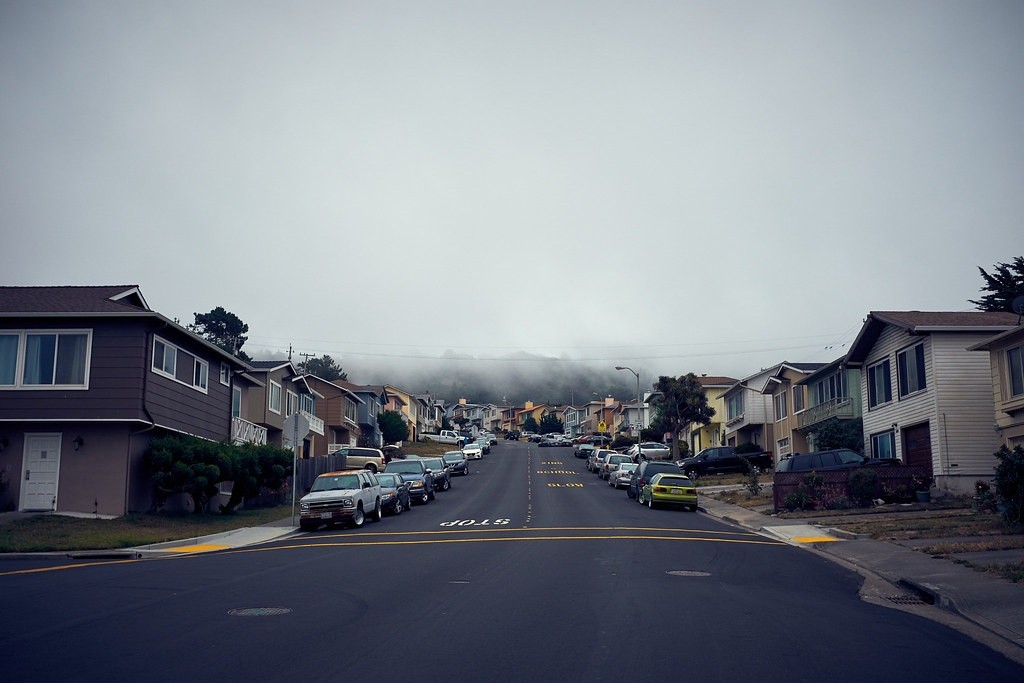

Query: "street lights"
<box><xmin>615</xmin><ymin>366</ymin><xmax>640</xmax><ymax>463</ymax></box>
<box><xmin>502</xmin><ymin>399</ymin><xmax>511</xmax><ymax>429</ymax></box>
<box><xmin>592</xmin><ymin>392</ymin><xmax>604</xmax><ymax>448</ymax></box>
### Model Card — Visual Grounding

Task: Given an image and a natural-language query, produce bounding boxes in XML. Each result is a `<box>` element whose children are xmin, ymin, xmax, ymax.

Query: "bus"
<box><xmin>770</xmin><ymin>448</ymin><xmax>906</xmax><ymax>470</ymax></box>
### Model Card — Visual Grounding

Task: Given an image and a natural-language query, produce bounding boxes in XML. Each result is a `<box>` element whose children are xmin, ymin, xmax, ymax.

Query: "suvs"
<box><xmin>627</xmin><ymin>441</ymin><xmax>671</xmax><ymax>463</ymax></box>
<box><xmin>627</xmin><ymin>462</ymin><xmax>683</xmax><ymax>502</ymax></box>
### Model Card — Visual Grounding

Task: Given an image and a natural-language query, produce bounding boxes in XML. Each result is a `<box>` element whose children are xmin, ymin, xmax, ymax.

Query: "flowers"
<box><xmin>908</xmin><ymin>471</ymin><xmax>935</xmax><ymax>491</ymax></box>
<box><xmin>805</xmin><ymin>472</ymin><xmax>827</xmax><ymax>501</ymax></box>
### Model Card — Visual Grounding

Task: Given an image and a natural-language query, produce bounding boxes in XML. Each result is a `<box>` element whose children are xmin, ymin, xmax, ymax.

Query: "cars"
<box><xmin>504</xmin><ymin>431</ymin><xmax>519</xmax><ymax>441</ymax></box>
<box><xmin>417</xmin><ymin>456</ymin><xmax>450</xmax><ymax>491</ymax></box>
<box><xmin>639</xmin><ymin>472</ymin><xmax>698</xmax><ymax>513</ymax></box>
<box><xmin>374</xmin><ymin>472</ymin><xmax>411</xmax><ymax>515</ymax></box>
<box><xmin>598</xmin><ymin>454</ymin><xmax>634</xmax><ymax>479</ymax></box>
<box><xmin>442</xmin><ymin>450</ymin><xmax>469</xmax><ymax>476</ymax></box>
<box><xmin>380</xmin><ymin>445</ymin><xmax>408</xmax><ymax>463</ymax></box>
<box><xmin>461</xmin><ymin>429</ymin><xmax>498</xmax><ymax>460</ymax></box>
<box><xmin>574</xmin><ymin>444</ymin><xmax>594</xmax><ymax>458</ymax></box>
<box><xmin>521</xmin><ymin>431</ymin><xmax>613</xmax><ymax>447</ymax></box>
<box><xmin>608</xmin><ymin>462</ymin><xmax>640</xmax><ymax>489</ymax></box>
<box><xmin>384</xmin><ymin>459</ymin><xmax>436</xmax><ymax>505</ymax></box>
<box><xmin>586</xmin><ymin>449</ymin><xmax>619</xmax><ymax>473</ymax></box>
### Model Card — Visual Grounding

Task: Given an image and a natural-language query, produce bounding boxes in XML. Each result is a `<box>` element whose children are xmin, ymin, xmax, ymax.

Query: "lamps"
<box><xmin>72</xmin><ymin>435</ymin><xmax>83</xmax><ymax>452</ymax></box>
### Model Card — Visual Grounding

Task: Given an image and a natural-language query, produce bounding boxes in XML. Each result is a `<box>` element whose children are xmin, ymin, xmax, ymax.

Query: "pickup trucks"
<box><xmin>417</xmin><ymin>429</ymin><xmax>474</xmax><ymax>447</ymax></box>
<box><xmin>298</xmin><ymin>470</ymin><xmax>382</xmax><ymax>530</ymax></box>
<box><xmin>675</xmin><ymin>447</ymin><xmax>774</xmax><ymax>476</ymax></box>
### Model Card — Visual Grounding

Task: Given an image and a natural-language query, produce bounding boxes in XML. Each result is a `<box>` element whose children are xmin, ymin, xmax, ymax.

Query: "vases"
<box><xmin>916</xmin><ymin>491</ymin><xmax>930</xmax><ymax>503</ymax></box>
<box><xmin>810</xmin><ymin>499</ymin><xmax>824</xmax><ymax>510</ymax></box>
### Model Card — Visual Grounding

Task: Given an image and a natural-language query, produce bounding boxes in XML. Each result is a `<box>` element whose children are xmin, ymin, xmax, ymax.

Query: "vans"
<box><xmin>331</xmin><ymin>446</ymin><xmax>387</xmax><ymax>474</ymax></box>
